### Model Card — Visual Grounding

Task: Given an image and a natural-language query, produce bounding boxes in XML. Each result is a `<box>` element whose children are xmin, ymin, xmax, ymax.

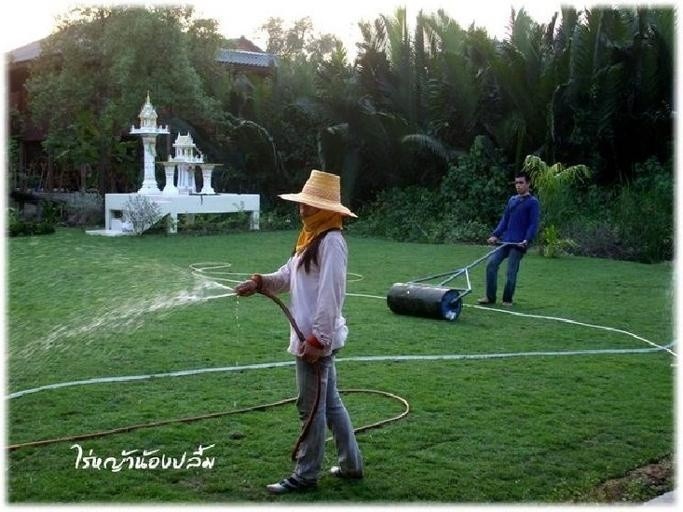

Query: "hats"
<box><xmin>276</xmin><ymin>169</ymin><xmax>359</xmax><ymax>218</ymax></box>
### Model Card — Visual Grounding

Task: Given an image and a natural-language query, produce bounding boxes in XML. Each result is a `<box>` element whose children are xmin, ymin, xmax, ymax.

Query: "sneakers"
<box><xmin>328</xmin><ymin>465</ymin><xmax>363</xmax><ymax>481</ymax></box>
<box><xmin>265</xmin><ymin>475</ymin><xmax>318</xmax><ymax>495</ymax></box>
<box><xmin>476</xmin><ymin>295</ymin><xmax>512</xmax><ymax>309</ymax></box>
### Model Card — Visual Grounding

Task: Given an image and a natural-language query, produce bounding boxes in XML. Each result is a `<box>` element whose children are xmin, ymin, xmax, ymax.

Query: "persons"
<box><xmin>476</xmin><ymin>171</ymin><xmax>539</xmax><ymax>307</ymax></box>
<box><xmin>232</xmin><ymin>168</ymin><xmax>363</xmax><ymax>495</ymax></box>
